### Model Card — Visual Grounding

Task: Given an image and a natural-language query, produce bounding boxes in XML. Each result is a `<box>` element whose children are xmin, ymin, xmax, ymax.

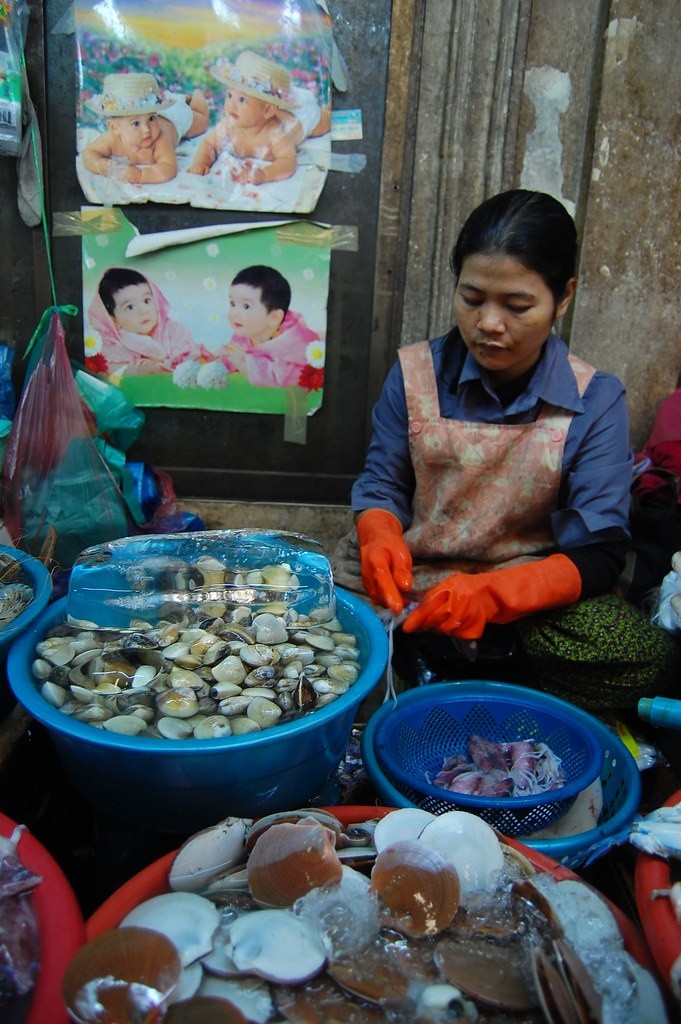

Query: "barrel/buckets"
<box><xmin>6</xmin><ymin>585</ymin><xmax>395</xmax><ymax>821</ymax></box>
<box><xmin>0</xmin><ymin>544</ymin><xmax>54</xmax><ymax>647</ymax></box>
<box><xmin>0</xmin><ymin>791</ymin><xmax>681</xmax><ymax>1024</ymax></box>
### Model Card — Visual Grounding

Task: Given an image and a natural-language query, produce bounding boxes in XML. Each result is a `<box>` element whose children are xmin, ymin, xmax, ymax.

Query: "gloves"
<box><xmin>356</xmin><ymin>508</ymin><xmax>413</xmax><ymax>617</ymax></box>
<box><xmin>402</xmin><ymin>552</ymin><xmax>582</xmax><ymax>639</ymax></box>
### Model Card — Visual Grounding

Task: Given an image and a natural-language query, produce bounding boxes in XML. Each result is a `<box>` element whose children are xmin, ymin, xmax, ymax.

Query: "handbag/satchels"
<box><xmin>1</xmin><ymin>309</ymin><xmax>205</xmax><ymax>602</ymax></box>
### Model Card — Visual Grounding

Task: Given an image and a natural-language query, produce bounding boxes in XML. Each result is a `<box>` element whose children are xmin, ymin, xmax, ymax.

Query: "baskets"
<box><xmin>364</xmin><ymin>679</ymin><xmax>640</xmax><ymax>870</ymax></box>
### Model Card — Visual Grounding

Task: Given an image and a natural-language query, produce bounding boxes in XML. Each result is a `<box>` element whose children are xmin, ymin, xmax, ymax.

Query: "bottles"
<box><xmin>638</xmin><ymin>696</ymin><xmax>681</xmax><ymax>731</ymax></box>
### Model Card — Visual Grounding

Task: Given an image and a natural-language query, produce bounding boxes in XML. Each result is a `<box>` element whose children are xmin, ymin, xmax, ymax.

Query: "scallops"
<box><xmin>34</xmin><ymin>559</ymin><xmax>358</xmax><ymax>741</ymax></box>
<box><xmin>61</xmin><ymin>808</ymin><xmax>665</xmax><ymax>1024</ymax></box>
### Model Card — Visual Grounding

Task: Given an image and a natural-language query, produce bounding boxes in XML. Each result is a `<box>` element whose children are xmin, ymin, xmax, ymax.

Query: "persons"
<box><xmin>626</xmin><ymin>370</ymin><xmax>681</xmax><ymax>614</ymax></box>
<box><xmin>82</xmin><ymin>72</ymin><xmax>208</xmax><ymax>184</ymax></box>
<box><xmin>352</xmin><ymin>190</ymin><xmax>632</xmax><ymax>692</ymax></box>
<box><xmin>219</xmin><ymin>265</ymin><xmax>325</xmax><ymax>387</ymax></box>
<box><xmin>89</xmin><ymin>267</ymin><xmax>215</xmax><ymax>374</ymax></box>
<box><xmin>186</xmin><ymin>51</ymin><xmax>331</xmax><ymax>185</ymax></box>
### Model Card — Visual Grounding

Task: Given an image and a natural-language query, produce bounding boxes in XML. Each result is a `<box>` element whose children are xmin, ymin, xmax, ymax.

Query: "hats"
<box><xmin>210</xmin><ymin>50</ymin><xmax>304</xmax><ymax>110</ymax></box>
<box><xmin>85</xmin><ymin>73</ymin><xmax>178</xmax><ymax>116</ymax></box>
